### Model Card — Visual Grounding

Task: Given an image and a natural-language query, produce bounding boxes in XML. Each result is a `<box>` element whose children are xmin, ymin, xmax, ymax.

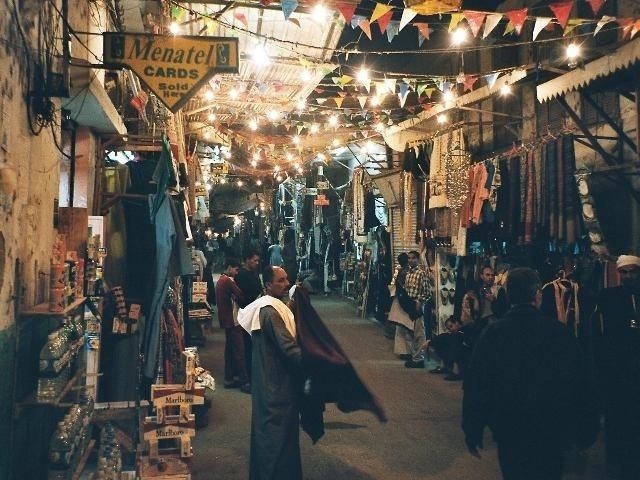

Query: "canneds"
<box><xmin>49</xmin><ymin>260</ymin><xmax>78</xmax><ymax>312</ymax></box>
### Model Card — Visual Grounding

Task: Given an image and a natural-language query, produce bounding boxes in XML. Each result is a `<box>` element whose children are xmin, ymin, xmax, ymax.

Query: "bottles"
<box><xmin>46</xmin><ymin>393</ymin><xmax>95</xmax><ymax>480</ymax></box>
<box><xmin>33</xmin><ymin>315</ymin><xmax>87</xmax><ymax>402</ymax></box>
<box><xmin>94</xmin><ymin>420</ymin><xmax>123</xmax><ymax>480</ymax></box>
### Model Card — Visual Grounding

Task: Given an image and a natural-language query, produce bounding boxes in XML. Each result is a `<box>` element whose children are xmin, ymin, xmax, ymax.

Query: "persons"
<box><xmin>236</xmin><ymin>266</ymin><xmax>387</xmax><ymax>480</ymax></box>
<box><xmin>211</xmin><ymin>231</ymin><xmax>325</xmax><ymax>393</ymax></box>
<box><xmin>387</xmin><ymin>249</ymin><xmax>640</xmax><ymax>479</ymax></box>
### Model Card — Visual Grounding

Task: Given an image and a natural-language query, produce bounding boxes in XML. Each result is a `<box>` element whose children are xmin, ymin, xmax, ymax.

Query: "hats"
<box><xmin>617</xmin><ymin>255</ymin><xmax>640</xmax><ymax>269</ymax></box>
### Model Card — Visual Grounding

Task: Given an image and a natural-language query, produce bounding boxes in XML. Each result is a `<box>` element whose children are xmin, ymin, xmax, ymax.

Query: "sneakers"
<box><xmin>404</xmin><ymin>360</ymin><xmax>424</xmax><ymax>368</ymax></box>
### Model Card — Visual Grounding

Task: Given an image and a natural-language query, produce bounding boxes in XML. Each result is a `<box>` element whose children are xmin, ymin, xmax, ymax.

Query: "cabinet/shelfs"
<box><xmin>12</xmin><ymin>257</ymin><xmax>87</xmax><ymax>420</ymax></box>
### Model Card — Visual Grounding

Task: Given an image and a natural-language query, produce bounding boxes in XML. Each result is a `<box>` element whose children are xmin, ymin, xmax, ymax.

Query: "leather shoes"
<box><xmin>431</xmin><ymin>367</ymin><xmax>446</xmax><ymax>373</ymax></box>
<box><xmin>444</xmin><ymin>374</ymin><xmax>462</xmax><ymax>381</ymax></box>
